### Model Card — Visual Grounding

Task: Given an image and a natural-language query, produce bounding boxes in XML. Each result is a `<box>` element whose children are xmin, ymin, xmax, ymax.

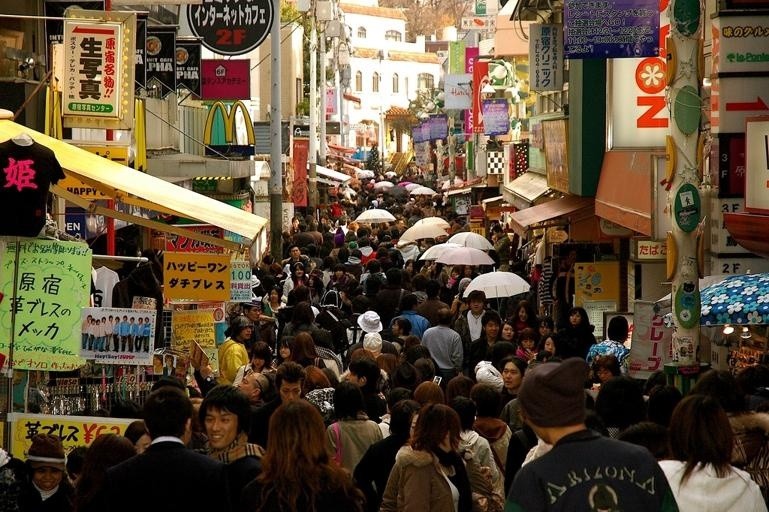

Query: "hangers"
<box><xmin>12</xmin><ymin>130</ymin><xmax>34</xmax><ymax>146</ymax></box>
<box><xmin>136</xmin><ymin>258</ymin><xmax>143</xmax><ymax>268</ymax></box>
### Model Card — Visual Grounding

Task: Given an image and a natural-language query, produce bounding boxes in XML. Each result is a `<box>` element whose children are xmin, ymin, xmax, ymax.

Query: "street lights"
<box><xmin>433</xmin><ymin>88</ymin><xmax>447</xmax><ymax>106</ymax></box>
<box><xmin>489</xmin><ymin>59</ymin><xmax>516</xmax><ymax>88</ymax></box>
<box><xmin>426</xmin><ymin>98</ymin><xmax>444</xmax><ymax>116</ymax></box>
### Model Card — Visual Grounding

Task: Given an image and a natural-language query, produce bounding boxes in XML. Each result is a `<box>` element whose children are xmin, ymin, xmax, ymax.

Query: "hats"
<box><xmin>362</xmin><ymin>331</ymin><xmax>384</xmax><ymax>360</ymax></box>
<box><xmin>516</xmin><ymin>356</ymin><xmax>592</xmax><ymax>429</ymax></box>
<box><xmin>357</xmin><ymin>309</ymin><xmax>384</xmax><ymax>335</ymax></box>
<box><xmin>244</xmin><ymin>295</ymin><xmax>263</xmax><ymax>308</ymax></box>
<box><xmin>25</xmin><ymin>432</ymin><xmax>67</xmax><ymax>465</ymax></box>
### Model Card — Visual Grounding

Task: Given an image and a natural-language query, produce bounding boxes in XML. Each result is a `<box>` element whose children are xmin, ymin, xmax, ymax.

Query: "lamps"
<box><xmin>19</xmin><ymin>57</ymin><xmax>35</xmax><ymax>78</ymax></box>
<box><xmin>723</xmin><ymin>324</ymin><xmax>735</xmax><ymax>334</ymax></box>
<box><xmin>739</xmin><ymin>326</ymin><xmax>752</xmax><ymax>339</ymax></box>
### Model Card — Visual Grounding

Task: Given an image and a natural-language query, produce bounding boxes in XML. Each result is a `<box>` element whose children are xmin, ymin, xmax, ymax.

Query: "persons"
<box><xmin>196</xmin><ymin>171</ymin><xmax>556</xmax><ymax>511</ymax></box>
<box><xmin>1</xmin><ymin>378</ymin><xmax>222</xmax><ymax>512</ymax></box>
<box><xmin>154</xmin><ymin>354</ymin><xmax>163</xmax><ymax>373</ymax></box>
<box><xmin>163</xmin><ymin>355</ymin><xmax>175</xmax><ymax>376</ymax></box>
<box><xmin>82</xmin><ymin>315</ymin><xmax>151</xmax><ymax>353</ymax></box>
<box><xmin>506</xmin><ymin>309</ymin><xmax>768</xmax><ymax>511</ymax></box>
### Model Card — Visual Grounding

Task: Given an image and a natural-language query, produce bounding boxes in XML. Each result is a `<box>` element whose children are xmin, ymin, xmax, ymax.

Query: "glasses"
<box><xmin>515</xmin><ymin>407</ymin><xmax>524</xmax><ymax>419</ymax></box>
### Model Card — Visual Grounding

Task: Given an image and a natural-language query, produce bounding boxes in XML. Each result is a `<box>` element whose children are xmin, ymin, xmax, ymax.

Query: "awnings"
<box><xmin>307</xmin><ymin>164</ymin><xmax>352</xmax><ymax>186</ymax></box>
<box><xmin>503</xmin><ymin>171</ymin><xmax>551</xmax><ymax>212</ymax></box>
<box><xmin>509</xmin><ymin>197</ymin><xmax>596</xmax><ymax>240</ymax></box>
<box><xmin>0</xmin><ymin>117</ymin><xmax>270</xmax><ymax>261</ymax></box>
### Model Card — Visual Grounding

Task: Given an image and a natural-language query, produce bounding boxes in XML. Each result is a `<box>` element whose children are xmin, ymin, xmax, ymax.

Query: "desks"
<box><xmin>663</xmin><ymin>362</ymin><xmax>711</xmax><ymax>395</ymax></box>
